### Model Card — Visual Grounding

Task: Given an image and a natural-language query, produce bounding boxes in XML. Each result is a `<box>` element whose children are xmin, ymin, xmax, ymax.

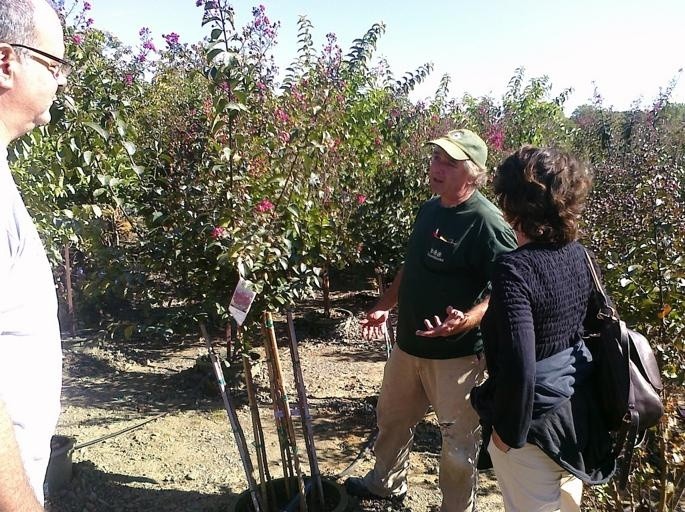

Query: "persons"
<box><xmin>343</xmin><ymin>128</ymin><xmax>522</xmax><ymax>512</ymax></box>
<box><xmin>0</xmin><ymin>0</ymin><xmax>69</xmax><ymax>512</ymax></box>
<box><xmin>470</xmin><ymin>142</ymin><xmax>623</xmax><ymax>512</ymax></box>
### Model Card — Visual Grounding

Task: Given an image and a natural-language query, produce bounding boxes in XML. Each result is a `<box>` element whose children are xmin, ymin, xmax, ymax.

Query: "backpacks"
<box><xmin>572</xmin><ymin>247</ymin><xmax>664</xmax><ymax>432</ymax></box>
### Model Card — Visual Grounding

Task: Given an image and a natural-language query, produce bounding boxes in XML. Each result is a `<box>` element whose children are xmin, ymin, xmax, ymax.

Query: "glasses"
<box><xmin>11</xmin><ymin>43</ymin><xmax>75</xmax><ymax>80</ymax></box>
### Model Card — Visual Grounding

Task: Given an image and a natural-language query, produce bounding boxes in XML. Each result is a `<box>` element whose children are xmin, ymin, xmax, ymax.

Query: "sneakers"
<box><xmin>345</xmin><ymin>477</ymin><xmax>407</xmax><ymax>501</ymax></box>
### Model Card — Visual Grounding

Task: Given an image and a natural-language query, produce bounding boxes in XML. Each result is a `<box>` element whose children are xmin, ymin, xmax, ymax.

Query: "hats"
<box><xmin>426</xmin><ymin>129</ymin><xmax>488</xmax><ymax>170</ymax></box>
